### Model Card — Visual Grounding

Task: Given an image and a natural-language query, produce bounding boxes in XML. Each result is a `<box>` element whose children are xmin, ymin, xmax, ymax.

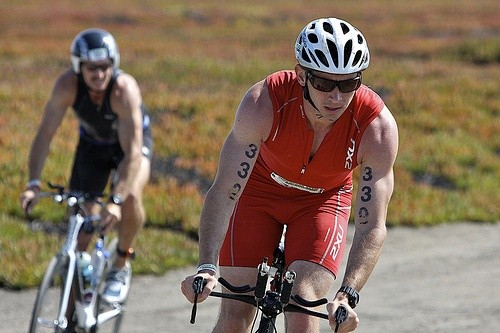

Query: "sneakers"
<box><xmin>102</xmin><ymin>265</ymin><xmax>135</xmax><ymax>302</ymax></box>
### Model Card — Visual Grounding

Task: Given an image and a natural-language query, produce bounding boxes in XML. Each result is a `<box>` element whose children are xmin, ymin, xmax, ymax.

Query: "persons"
<box><xmin>18</xmin><ymin>28</ymin><xmax>152</xmax><ymax>302</ymax></box>
<box><xmin>181</xmin><ymin>17</ymin><xmax>399</xmax><ymax>333</ymax></box>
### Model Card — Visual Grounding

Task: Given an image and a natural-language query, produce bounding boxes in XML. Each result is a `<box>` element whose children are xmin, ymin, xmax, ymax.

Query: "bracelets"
<box><xmin>197</xmin><ymin>263</ymin><xmax>216</xmax><ymax>273</ymax></box>
<box><xmin>27</xmin><ymin>179</ymin><xmax>40</xmax><ymax>188</ymax></box>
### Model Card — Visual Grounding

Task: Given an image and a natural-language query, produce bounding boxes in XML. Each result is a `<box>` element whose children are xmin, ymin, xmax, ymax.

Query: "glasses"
<box><xmin>84</xmin><ymin>63</ymin><xmax>111</xmax><ymax>72</ymax></box>
<box><xmin>308</xmin><ymin>70</ymin><xmax>362</xmax><ymax>93</ymax></box>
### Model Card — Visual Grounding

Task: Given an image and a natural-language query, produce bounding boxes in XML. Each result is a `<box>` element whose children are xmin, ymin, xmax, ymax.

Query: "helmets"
<box><xmin>292</xmin><ymin>16</ymin><xmax>370</xmax><ymax>75</ymax></box>
<box><xmin>68</xmin><ymin>29</ymin><xmax>122</xmax><ymax>73</ymax></box>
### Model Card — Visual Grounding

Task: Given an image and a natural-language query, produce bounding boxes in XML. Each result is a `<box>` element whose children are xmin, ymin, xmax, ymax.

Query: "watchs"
<box><xmin>338</xmin><ymin>286</ymin><xmax>359</xmax><ymax>308</ymax></box>
<box><xmin>110</xmin><ymin>193</ymin><xmax>122</xmax><ymax>205</ymax></box>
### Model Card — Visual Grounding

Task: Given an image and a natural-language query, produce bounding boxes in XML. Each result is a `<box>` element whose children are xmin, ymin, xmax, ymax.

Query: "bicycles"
<box><xmin>190</xmin><ymin>245</ymin><xmax>348</xmax><ymax>333</ymax></box>
<box><xmin>25</xmin><ymin>181</ymin><xmax>126</xmax><ymax>333</ymax></box>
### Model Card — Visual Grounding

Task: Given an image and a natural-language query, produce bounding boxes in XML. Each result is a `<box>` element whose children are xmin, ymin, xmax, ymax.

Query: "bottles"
<box><xmin>91</xmin><ymin>243</ymin><xmax>105</xmax><ymax>287</ymax></box>
<box><xmin>77</xmin><ymin>252</ymin><xmax>94</xmax><ymax>288</ymax></box>
